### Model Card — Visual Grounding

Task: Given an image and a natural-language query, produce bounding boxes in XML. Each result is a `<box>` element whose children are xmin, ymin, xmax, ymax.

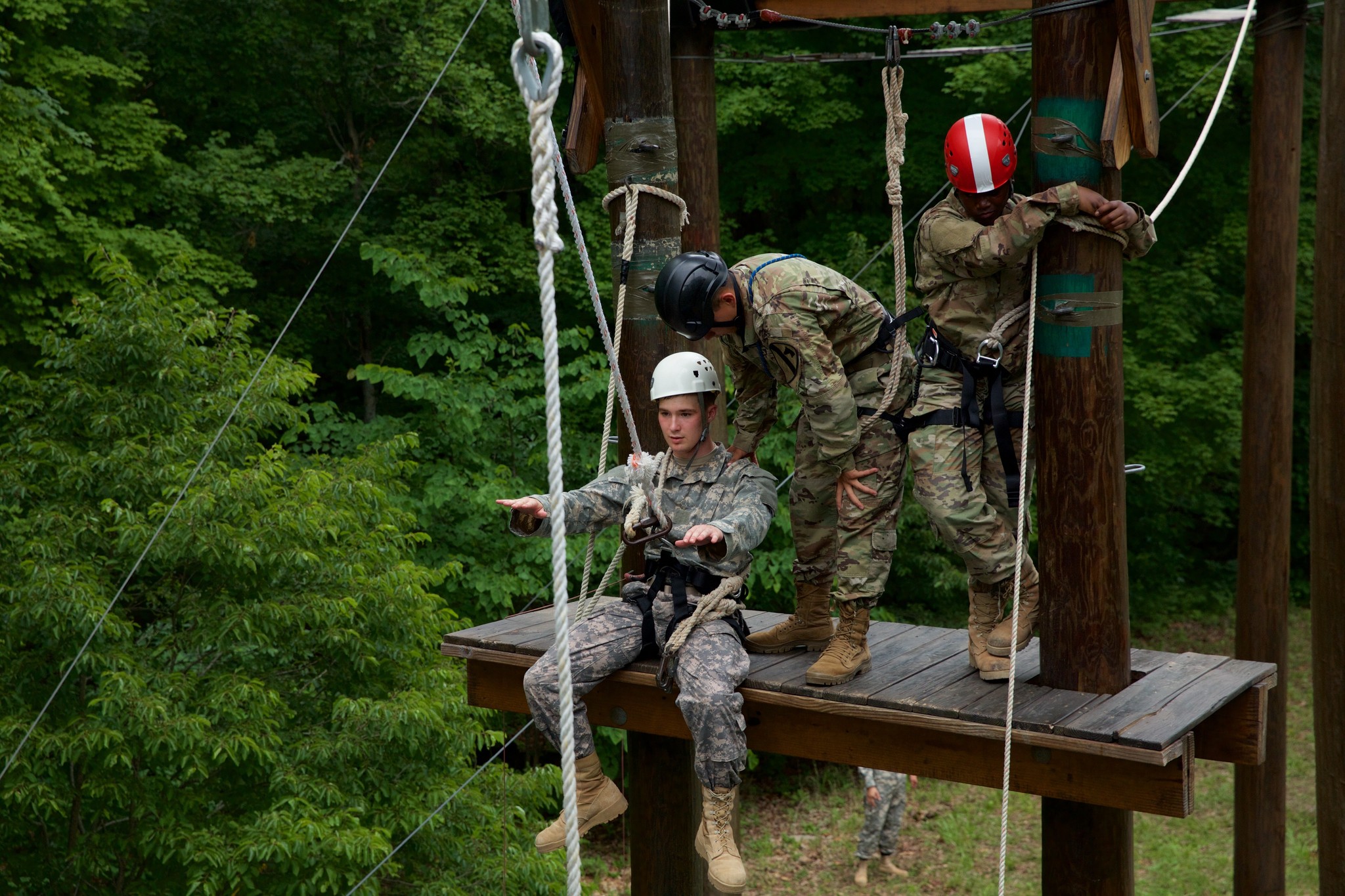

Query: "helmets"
<box><xmin>649</xmin><ymin>351</ymin><xmax>722</xmax><ymax>401</ymax></box>
<box><xmin>944</xmin><ymin>114</ymin><xmax>1018</xmax><ymax>193</ymax></box>
<box><xmin>654</xmin><ymin>250</ymin><xmax>728</xmax><ymax>339</ymax></box>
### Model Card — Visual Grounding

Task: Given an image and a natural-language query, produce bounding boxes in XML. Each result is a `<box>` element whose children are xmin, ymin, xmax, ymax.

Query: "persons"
<box><xmin>854</xmin><ymin>766</ymin><xmax>918</xmax><ymax>885</ymax></box>
<box><xmin>654</xmin><ymin>249</ymin><xmax>917</xmax><ymax>687</ymax></box>
<box><xmin>900</xmin><ymin>111</ymin><xmax>1159</xmax><ymax>685</ymax></box>
<box><xmin>495</xmin><ymin>350</ymin><xmax>779</xmax><ymax>894</ymax></box>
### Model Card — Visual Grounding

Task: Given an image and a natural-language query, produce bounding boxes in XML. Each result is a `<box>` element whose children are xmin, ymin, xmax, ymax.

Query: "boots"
<box><xmin>742</xmin><ymin>580</ymin><xmax>834</xmax><ymax>654</ymax></box>
<box><xmin>879</xmin><ymin>855</ymin><xmax>909</xmax><ymax>878</ymax></box>
<box><xmin>967</xmin><ymin>578</ymin><xmax>1013</xmax><ymax>680</ymax></box>
<box><xmin>986</xmin><ymin>552</ymin><xmax>1041</xmax><ymax>655</ymax></box>
<box><xmin>854</xmin><ymin>860</ymin><xmax>869</xmax><ymax>886</ymax></box>
<box><xmin>805</xmin><ymin>599</ymin><xmax>871</xmax><ymax>684</ymax></box>
<box><xmin>694</xmin><ymin>783</ymin><xmax>746</xmax><ymax>892</ymax></box>
<box><xmin>534</xmin><ymin>750</ymin><xmax>628</xmax><ymax>853</ymax></box>
